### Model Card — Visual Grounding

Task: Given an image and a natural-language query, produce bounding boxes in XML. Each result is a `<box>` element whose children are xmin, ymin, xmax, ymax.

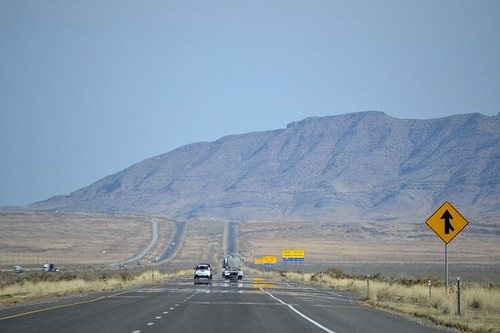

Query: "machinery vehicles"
<box><xmin>221</xmin><ymin>253</ymin><xmax>245</xmax><ymax>279</ymax></box>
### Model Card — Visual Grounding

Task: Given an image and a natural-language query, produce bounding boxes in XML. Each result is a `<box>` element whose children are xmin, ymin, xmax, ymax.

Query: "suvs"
<box><xmin>193</xmin><ymin>263</ymin><xmax>212</xmax><ymax>279</ymax></box>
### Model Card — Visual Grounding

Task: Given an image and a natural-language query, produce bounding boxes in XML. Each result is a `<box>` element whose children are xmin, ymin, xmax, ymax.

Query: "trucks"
<box><xmin>41</xmin><ymin>264</ymin><xmax>55</xmax><ymax>272</ymax></box>
